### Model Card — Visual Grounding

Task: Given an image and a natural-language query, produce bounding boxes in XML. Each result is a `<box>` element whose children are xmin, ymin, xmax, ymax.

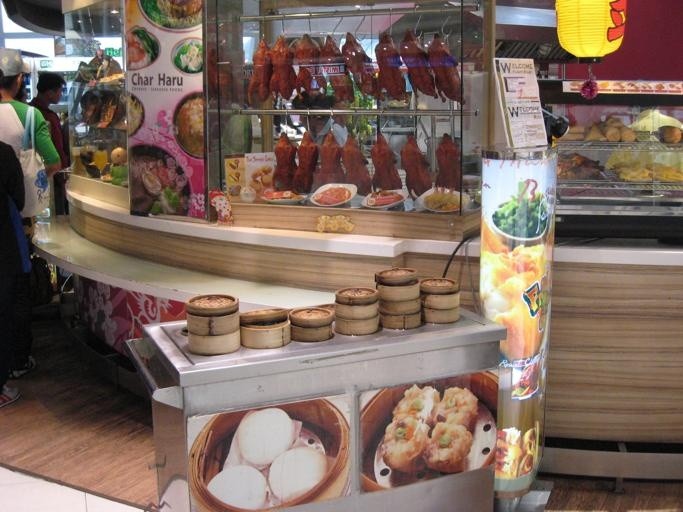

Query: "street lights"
<box><xmin>19</xmin><ymin>106</ymin><xmax>51</xmax><ymax>218</ymax></box>
<box><xmin>29</xmin><ymin>255</ymin><xmax>54</xmax><ymax>309</ymax></box>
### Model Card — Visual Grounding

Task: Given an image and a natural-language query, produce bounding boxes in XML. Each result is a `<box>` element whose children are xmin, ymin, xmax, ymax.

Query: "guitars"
<box><xmin>554</xmin><ymin>0</ymin><xmax>627</xmax><ymax>100</ymax></box>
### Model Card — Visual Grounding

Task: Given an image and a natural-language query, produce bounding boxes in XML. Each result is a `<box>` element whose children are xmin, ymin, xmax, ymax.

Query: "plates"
<box><xmin>535</xmin><ymin>78</ymin><xmax>683</xmax><ymax>239</ymax></box>
<box><xmin>201</xmin><ymin>2</ymin><xmax>494</xmax><ymax>244</ymax></box>
<box><xmin>69</xmin><ymin>81</ymin><xmax>129</xmax><ymax>209</ymax></box>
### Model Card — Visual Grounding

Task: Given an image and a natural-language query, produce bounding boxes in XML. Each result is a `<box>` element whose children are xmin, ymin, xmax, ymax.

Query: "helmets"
<box><xmin>361</xmin><ymin>190</ymin><xmax>409</xmax><ymax>210</ymax></box>
<box><xmin>309</xmin><ymin>183</ymin><xmax>358</xmax><ymax>207</ymax></box>
<box><xmin>261</xmin><ymin>192</ymin><xmax>310</xmax><ymax>205</ymax></box>
<box><xmin>416</xmin><ymin>186</ymin><xmax>470</xmax><ymax>213</ymax></box>
<box><xmin>127</xmin><ymin>1</ymin><xmax>203</xmax><ymax>75</ymax></box>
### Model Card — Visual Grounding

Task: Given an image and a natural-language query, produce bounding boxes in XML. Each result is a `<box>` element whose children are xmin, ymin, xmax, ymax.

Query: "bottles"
<box><xmin>0</xmin><ymin>48</ymin><xmax>31</xmax><ymax>77</ymax></box>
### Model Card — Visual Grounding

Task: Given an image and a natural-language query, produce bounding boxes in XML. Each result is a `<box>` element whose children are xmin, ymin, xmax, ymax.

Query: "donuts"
<box><xmin>290</xmin><ymin>131</ymin><xmax>319</xmax><ymax>193</ymax></box>
<box><xmin>314</xmin><ymin>130</ymin><xmax>345</xmax><ymax>184</ymax></box>
<box><xmin>248</xmin><ymin>29</ymin><xmax>466</xmax><ymax>105</ymax></box>
<box><xmin>435</xmin><ymin>133</ymin><xmax>461</xmax><ymax>191</ymax></box>
<box><xmin>371</xmin><ymin>132</ymin><xmax>403</xmax><ymax>192</ymax></box>
<box><xmin>401</xmin><ymin>134</ymin><xmax>432</xmax><ymax>201</ymax></box>
<box><xmin>272</xmin><ymin>132</ymin><xmax>298</xmax><ymax>192</ymax></box>
<box><xmin>340</xmin><ymin>134</ymin><xmax>373</xmax><ymax>197</ymax></box>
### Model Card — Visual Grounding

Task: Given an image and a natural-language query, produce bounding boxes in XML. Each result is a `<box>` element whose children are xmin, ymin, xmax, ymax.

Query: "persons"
<box><xmin>0</xmin><ymin>48</ymin><xmax>68</xmax><ymax>408</ymax></box>
<box><xmin>293</xmin><ymin>88</ymin><xmax>348</xmax><ymax>175</ymax></box>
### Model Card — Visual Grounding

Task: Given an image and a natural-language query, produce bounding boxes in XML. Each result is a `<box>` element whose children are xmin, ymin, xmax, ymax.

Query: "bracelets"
<box><xmin>125</xmin><ymin>90</ymin><xmax>205</xmax><ymax>216</ymax></box>
<box><xmin>490</xmin><ymin>200</ymin><xmax>549</xmax><ymax>250</ymax></box>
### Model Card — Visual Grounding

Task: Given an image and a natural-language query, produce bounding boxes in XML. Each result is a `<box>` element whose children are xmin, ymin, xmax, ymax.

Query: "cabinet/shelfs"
<box><xmin>33</xmin><ymin>207</ymin><xmax>50</xmax><ymax>245</ymax></box>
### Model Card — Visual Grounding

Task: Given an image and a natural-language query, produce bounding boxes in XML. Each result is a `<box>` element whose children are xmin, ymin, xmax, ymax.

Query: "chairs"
<box><xmin>0</xmin><ymin>384</ymin><xmax>21</xmax><ymax>408</ymax></box>
<box><xmin>10</xmin><ymin>356</ymin><xmax>37</xmax><ymax>379</ymax></box>
